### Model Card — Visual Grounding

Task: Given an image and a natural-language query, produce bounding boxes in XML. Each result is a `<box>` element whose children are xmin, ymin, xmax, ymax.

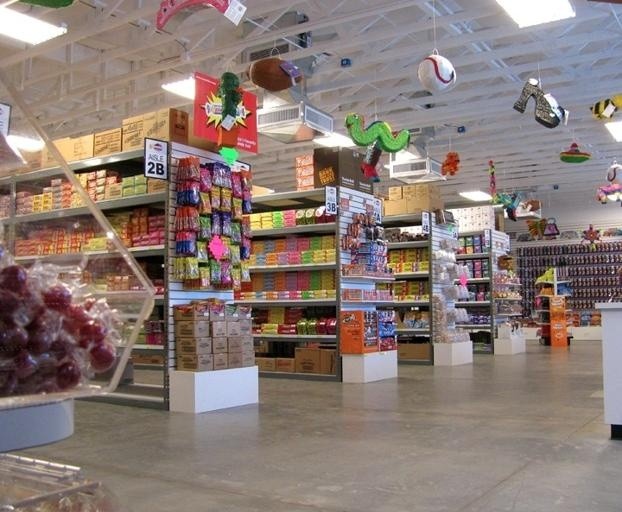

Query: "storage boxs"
<box><xmin>41</xmin><ymin>107</ymin><xmax>188</xmax><ymax>169</ymax></box>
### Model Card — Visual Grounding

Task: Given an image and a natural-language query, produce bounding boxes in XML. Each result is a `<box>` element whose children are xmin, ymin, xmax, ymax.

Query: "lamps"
<box><xmin>604</xmin><ymin>117</ymin><xmax>622</xmax><ymax>141</ymax></box>
<box><xmin>495</xmin><ymin>0</ymin><xmax>577</xmax><ymax>31</ymax></box>
<box><xmin>312</xmin><ymin>129</ymin><xmax>357</xmax><ymax>148</ymax></box>
<box><xmin>6</xmin><ymin>130</ymin><xmax>45</xmax><ymax>153</ymax></box>
<box><xmin>159</xmin><ymin>72</ymin><xmax>196</xmax><ymax>101</ymax></box>
<box><xmin>0</xmin><ymin>2</ymin><xmax>68</xmax><ymax>48</ymax></box>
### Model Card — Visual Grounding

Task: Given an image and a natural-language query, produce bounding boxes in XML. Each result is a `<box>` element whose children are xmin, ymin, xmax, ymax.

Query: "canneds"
<box><xmin>515</xmin><ymin>242</ymin><xmax>622</xmax><ymax>309</ymax></box>
